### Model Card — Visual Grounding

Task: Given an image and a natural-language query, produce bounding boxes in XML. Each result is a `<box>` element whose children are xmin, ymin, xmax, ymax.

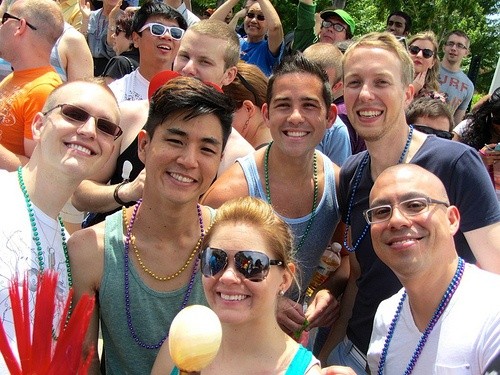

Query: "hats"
<box><xmin>320</xmin><ymin>8</ymin><xmax>356</xmax><ymax>38</ymax></box>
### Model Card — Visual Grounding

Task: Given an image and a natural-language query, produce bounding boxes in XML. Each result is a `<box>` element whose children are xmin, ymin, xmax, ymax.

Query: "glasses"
<box><xmin>362</xmin><ymin>197</ymin><xmax>450</xmax><ymax>225</ymax></box>
<box><xmin>322</xmin><ymin>19</ymin><xmax>348</xmax><ymax>32</ymax></box>
<box><xmin>408</xmin><ymin>45</ymin><xmax>437</xmax><ymax>59</ymax></box>
<box><xmin>245</xmin><ymin>12</ymin><xmax>266</xmax><ymax>20</ymax></box>
<box><xmin>411</xmin><ymin>124</ymin><xmax>455</xmax><ymax>142</ymax></box>
<box><xmin>114</xmin><ymin>26</ymin><xmax>128</xmax><ymax>36</ymax></box>
<box><xmin>1</xmin><ymin>11</ymin><xmax>38</xmax><ymax>32</ymax></box>
<box><xmin>138</xmin><ymin>22</ymin><xmax>185</xmax><ymax>41</ymax></box>
<box><xmin>199</xmin><ymin>246</ymin><xmax>287</xmax><ymax>283</ymax></box>
<box><xmin>42</xmin><ymin>103</ymin><xmax>124</xmax><ymax>141</ymax></box>
<box><xmin>445</xmin><ymin>41</ymin><xmax>468</xmax><ymax>49</ymax></box>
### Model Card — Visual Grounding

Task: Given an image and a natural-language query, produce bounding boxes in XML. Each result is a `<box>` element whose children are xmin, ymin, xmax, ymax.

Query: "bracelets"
<box><xmin>113</xmin><ymin>180</ymin><xmax>133</xmax><ymax>207</ymax></box>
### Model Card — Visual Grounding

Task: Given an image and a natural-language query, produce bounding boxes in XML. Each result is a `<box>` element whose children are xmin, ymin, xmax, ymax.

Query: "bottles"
<box><xmin>305</xmin><ymin>241</ymin><xmax>343</xmax><ymax>298</ymax></box>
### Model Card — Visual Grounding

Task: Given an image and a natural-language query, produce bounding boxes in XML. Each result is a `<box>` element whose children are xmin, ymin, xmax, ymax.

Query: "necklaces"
<box><xmin>19</xmin><ymin>166</ymin><xmax>73</xmax><ymax>340</ymax></box>
<box><xmin>377</xmin><ymin>259</ymin><xmax>465</xmax><ymax>375</ymax></box>
<box><xmin>125</xmin><ymin>199</ymin><xmax>205</xmax><ymax>350</ymax></box>
<box><xmin>343</xmin><ymin>123</ymin><xmax>414</xmax><ymax>251</ymax></box>
<box><xmin>265</xmin><ymin>140</ymin><xmax>318</xmax><ymax>252</ymax></box>
<box><xmin>121</xmin><ymin>205</ymin><xmax>214</xmax><ymax>281</ymax></box>
<box><xmin>378</xmin><ymin>255</ymin><xmax>462</xmax><ymax>375</ymax></box>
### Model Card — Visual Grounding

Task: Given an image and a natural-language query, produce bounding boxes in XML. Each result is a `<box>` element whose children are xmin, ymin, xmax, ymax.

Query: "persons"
<box><xmin>0</xmin><ymin>0</ymin><xmax>500</xmax><ymax>375</ymax></box>
